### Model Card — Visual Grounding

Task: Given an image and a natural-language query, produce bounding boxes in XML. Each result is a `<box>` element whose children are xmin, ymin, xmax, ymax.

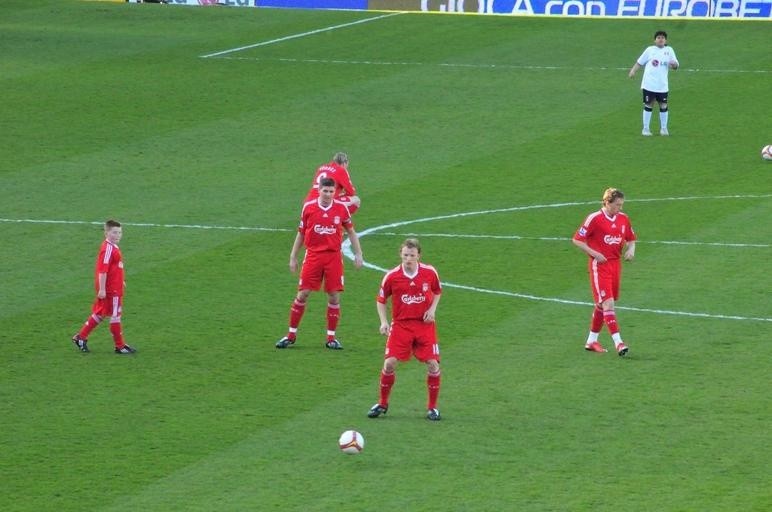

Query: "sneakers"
<box><xmin>326</xmin><ymin>339</ymin><xmax>343</xmax><ymax>350</ymax></box>
<box><xmin>660</xmin><ymin>128</ymin><xmax>668</xmax><ymax>136</ymax></box>
<box><xmin>368</xmin><ymin>404</ymin><xmax>388</xmax><ymax>417</ymax></box>
<box><xmin>72</xmin><ymin>332</ymin><xmax>90</xmax><ymax>352</ymax></box>
<box><xmin>114</xmin><ymin>344</ymin><xmax>135</xmax><ymax>354</ymax></box>
<box><xmin>617</xmin><ymin>342</ymin><xmax>628</xmax><ymax>356</ymax></box>
<box><xmin>585</xmin><ymin>341</ymin><xmax>606</xmax><ymax>353</ymax></box>
<box><xmin>427</xmin><ymin>408</ymin><xmax>440</xmax><ymax>420</ymax></box>
<box><xmin>275</xmin><ymin>337</ymin><xmax>296</xmax><ymax>347</ymax></box>
<box><xmin>642</xmin><ymin>128</ymin><xmax>652</xmax><ymax>136</ymax></box>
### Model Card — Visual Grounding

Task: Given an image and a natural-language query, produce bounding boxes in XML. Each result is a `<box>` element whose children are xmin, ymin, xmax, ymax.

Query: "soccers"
<box><xmin>340</xmin><ymin>430</ymin><xmax>364</xmax><ymax>456</ymax></box>
<box><xmin>762</xmin><ymin>145</ymin><xmax>772</xmax><ymax>160</ymax></box>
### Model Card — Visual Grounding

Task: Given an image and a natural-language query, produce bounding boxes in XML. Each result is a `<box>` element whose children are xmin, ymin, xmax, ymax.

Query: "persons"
<box><xmin>629</xmin><ymin>30</ymin><xmax>680</xmax><ymax>137</ymax></box>
<box><xmin>367</xmin><ymin>238</ymin><xmax>445</xmax><ymax>422</ymax></box>
<box><xmin>71</xmin><ymin>219</ymin><xmax>138</xmax><ymax>354</ymax></box>
<box><xmin>571</xmin><ymin>186</ymin><xmax>637</xmax><ymax>357</ymax></box>
<box><xmin>274</xmin><ymin>177</ymin><xmax>364</xmax><ymax>350</ymax></box>
<box><xmin>303</xmin><ymin>152</ymin><xmax>362</xmax><ymax>214</ymax></box>
